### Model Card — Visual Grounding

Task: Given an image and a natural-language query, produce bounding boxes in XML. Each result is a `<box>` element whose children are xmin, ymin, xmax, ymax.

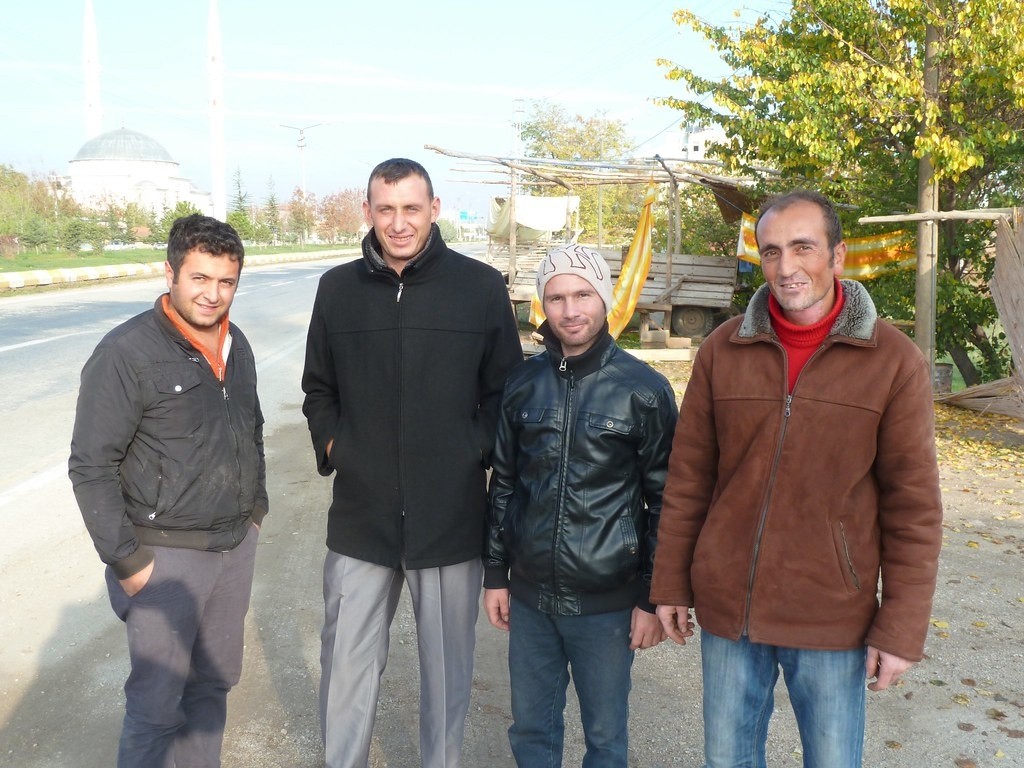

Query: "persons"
<box><xmin>300</xmin><ymin>158</ymin><xmax>525</xmax><ymax>768</ymax></box>
<box><xmin>483</xmin><ymin>246</ymin><xmax>679</xmax><ymax>768</ymax></box>
<box><xmin>649</xmin><ymin>188</ymin><xmax>944</xmax><ymax>768</ymax></box>
<box><xmin>69</xmin><ymin>215</ymin><xmax>269</xmax><ymax>768</ymax></box>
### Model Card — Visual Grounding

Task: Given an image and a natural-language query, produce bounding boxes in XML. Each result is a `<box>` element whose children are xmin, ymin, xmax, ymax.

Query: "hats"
<box><xmin>536</xmin><ymin>244</ymin><xmax>613</xmax><ymax>315</ymax></box>
